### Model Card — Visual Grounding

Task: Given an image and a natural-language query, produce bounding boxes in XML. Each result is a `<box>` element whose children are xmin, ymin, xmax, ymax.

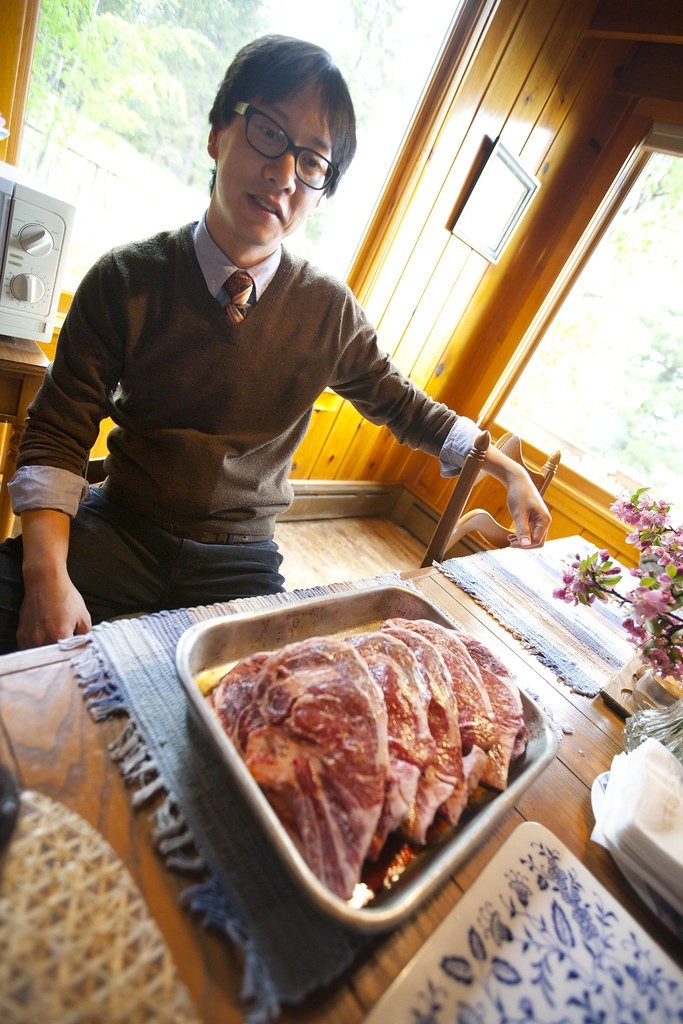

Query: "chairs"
<box><xmin>418</xmin><ymin>430</ymin><xmax>562</xmax><ymax>570</ymax></box>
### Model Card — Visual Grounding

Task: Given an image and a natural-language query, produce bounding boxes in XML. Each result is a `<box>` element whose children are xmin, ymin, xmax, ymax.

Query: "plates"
<box><xmin>341</xmin><ymin>819</ymin><xmax>682</xmax><ymax>1024</ymax></box>
<box><xmin>591</xmin><ymin>768</ymin><xmax>683</xmax><ymax>942</ymax></box>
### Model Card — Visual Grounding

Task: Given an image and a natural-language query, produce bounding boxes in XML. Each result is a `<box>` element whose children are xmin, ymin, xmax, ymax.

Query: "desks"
<box><xmin>0</xmin><ymin>334</ymin><xmax>53</xmax><ymax>546</ymax></box>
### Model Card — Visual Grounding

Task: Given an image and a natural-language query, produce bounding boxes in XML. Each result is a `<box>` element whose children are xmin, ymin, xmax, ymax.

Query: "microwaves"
<box><xmin>0</xmin><ymin>169</ymin><xmax>77</xmax><ymax>343</ymax></box>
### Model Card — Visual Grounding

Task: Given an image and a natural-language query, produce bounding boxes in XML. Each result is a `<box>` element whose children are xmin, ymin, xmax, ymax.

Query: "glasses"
<box><xmin>233</xmin><ymin>102</ymin><xmax>340</xmax><ymax>190</ymax></box>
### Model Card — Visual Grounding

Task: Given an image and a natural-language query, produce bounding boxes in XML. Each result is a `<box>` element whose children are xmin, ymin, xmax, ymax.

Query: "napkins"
<box><xmin>588</xmin><ymin>736</ymin><xmax>683</xmax><ymax>917</ymax></box>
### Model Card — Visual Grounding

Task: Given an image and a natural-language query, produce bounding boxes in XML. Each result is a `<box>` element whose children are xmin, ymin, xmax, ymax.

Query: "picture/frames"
<box><xmin>450</xmin><ymin>134</ymin><xmax>542</xmax><ymax>265</ymax></box>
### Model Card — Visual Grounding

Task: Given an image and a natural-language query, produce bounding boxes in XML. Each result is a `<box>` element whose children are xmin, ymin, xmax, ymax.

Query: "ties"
<box><xmin>225</xmin><ymin>273</ymin><xmax>254</xmax><ymax>324</ymax></box>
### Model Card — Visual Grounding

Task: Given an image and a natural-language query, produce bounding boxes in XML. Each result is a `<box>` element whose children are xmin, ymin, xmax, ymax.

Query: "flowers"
<box><xmin>551</xmin><ymin>486</ymin><xmax>683</xmax><ymax>683</ymax></box>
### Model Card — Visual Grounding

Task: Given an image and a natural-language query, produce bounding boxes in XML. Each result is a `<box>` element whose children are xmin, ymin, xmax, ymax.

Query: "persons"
<box><xmin>1</xmin><ymin>32</ymin><xmax>556</xmax><ymax>654</ymax></box>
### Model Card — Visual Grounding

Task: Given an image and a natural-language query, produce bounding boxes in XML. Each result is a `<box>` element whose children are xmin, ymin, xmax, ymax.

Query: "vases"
<box><xmin>622</xmin><ymin>696</ymin><xmax>683</xmax><ymax>766</ymax></box>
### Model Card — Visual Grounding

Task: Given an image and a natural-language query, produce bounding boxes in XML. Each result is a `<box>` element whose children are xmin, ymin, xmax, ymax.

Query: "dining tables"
<box><xmin>0</xmin><ymin>530</ymin><xmax>683</xmax><ymax>1024</ymax></box>
